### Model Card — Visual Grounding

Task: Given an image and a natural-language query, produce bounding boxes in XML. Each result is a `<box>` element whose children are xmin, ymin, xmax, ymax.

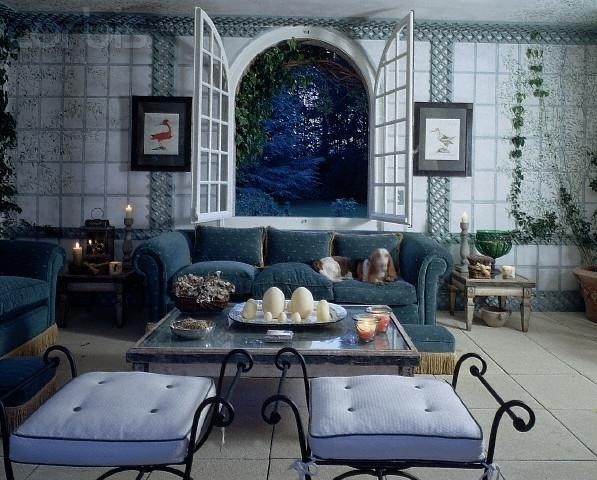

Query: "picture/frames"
<box><xmin>413</xmin><ymin>102</ymin><xmax>473</xmax><ymax>176</ymax></box>
<box><xmin>131</xmin><ymin>96</ymin><xmax>192</xmax><ymax>171</ymax></box>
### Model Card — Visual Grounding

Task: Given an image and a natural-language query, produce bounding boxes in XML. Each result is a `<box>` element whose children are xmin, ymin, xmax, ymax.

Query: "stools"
<box><xmin>401</xmin><ymin>324</ymin><xmax>456</xmax><ymax>374</ymax></box>
<box><xmin>0</xmin><ymin>358</ymin><xmax>59</xmax><ymax>435</ymax></box>
<box><xmin>261</xmin><ymin>346</ymin><xmax>535</xmax><ymax>480</ymax></box>
<box><xmin>0</xmin><ymin>345</ymin><xmax>253</xmax><ymax>480</ymax></box>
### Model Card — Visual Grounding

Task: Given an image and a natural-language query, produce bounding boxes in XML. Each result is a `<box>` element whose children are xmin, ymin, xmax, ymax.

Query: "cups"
<box><xmin>109</xmin><ymin>261</ymin><xmax>123</xmax><ymax>274</ymax></box>
<box><xmin>352</xmin><ymin>314</ymin><xmax>380</xmax><ymax>343</ymax></box>
<box><xmin>501</xmin><ymin>265</ymin><xmax>516</xmax><ymax>280</ymax></box>
<box><xmin>366</xmin><ymin>304</ymin><xmax>392</xmax><ymax>333</ymax></box>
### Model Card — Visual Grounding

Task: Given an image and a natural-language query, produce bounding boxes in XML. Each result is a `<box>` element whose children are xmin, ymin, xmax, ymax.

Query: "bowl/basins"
<box><xmin>169</xmin><ymin>319</ymin><xmax>217</xmax><ymax>339</ymax></box>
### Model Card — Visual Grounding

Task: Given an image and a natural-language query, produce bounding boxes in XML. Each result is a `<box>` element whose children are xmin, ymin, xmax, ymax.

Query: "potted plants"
<box><xmin>511</xmin><ymin>31</ymin><xmax>597</xmax><ymax>323</ymax></box>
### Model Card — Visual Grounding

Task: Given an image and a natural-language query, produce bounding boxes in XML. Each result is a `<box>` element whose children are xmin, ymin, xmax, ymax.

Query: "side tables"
<box><xmin>448</xmin><ymin>269</ymin><xmax>535</xmax><ymax>333</ymax></box>
<box><xmin>54</xmin><ymin>264</ymin><xmax>143</xmax><ymax>329</ymax></box>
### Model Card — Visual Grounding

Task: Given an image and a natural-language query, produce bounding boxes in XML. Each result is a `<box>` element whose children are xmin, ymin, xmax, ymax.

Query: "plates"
<box><xmin>228</xmin><ymin>300</ymin><xmax>348</xmax><ymax>325</ymax></box>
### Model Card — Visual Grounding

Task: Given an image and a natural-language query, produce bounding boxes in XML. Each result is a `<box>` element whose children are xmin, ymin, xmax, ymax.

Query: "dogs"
<box><xmin>310</xmin><ymin>248</ymin><xmax>397</xmax><ymax>288</ymax></box>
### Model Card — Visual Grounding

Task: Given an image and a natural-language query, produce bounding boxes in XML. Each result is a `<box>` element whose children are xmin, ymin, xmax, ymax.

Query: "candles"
<box><xmin>72</xmin><ymin>242</ymin><xmax>82</xmax><ymax>265</ymax></box>
<box><xmin>126</xmin><ymin>204</ymin><xmax>133</xmax><ymax>219</ymax></box>
<box><xmin>462</xmin><ymin>212</ymin><xmax>468</xmax><ymax>223</ymax></box>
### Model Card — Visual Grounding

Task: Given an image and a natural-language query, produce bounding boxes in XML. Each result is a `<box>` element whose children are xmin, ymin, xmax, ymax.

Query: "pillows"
<box><xmin>266</xmin><ymin>226</ymin><xmax>335</xmax><ymax>266</ymax></box>
<box><xmin>191</xmin><ymin>224</ymin><xmax>265</xmax><ymax>269</ymax></box>
<box><xmin>335</xmin><ymin>232</ymin><xmax>404</xmax><ymax>275</ymax></box>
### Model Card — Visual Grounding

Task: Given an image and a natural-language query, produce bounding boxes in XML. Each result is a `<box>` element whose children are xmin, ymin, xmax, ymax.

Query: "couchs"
<box><xmin>0</xmin><ymin>240</ymin><xmax>67</xmax><ymax>356</ymax></box>
<box><xmin>133</xmin><ymin>229</ymin><xmax>453</xmax><ymax>325</ymax></box>
